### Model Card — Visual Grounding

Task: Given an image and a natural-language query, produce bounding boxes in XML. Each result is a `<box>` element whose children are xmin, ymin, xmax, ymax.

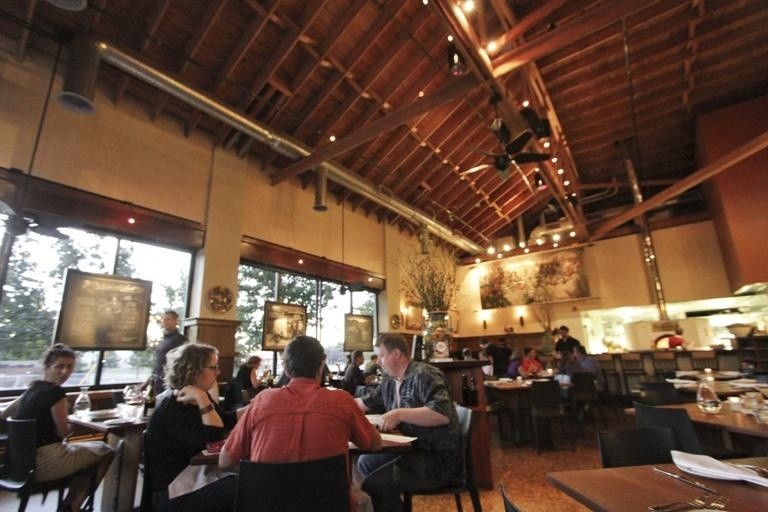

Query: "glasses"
<box><xmin>207</xmin><ymin>365</ymin><xmax>219</xmax><ymax>371</ymax></box>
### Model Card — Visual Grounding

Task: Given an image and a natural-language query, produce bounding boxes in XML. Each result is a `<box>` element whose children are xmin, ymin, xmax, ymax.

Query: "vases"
<box><xmin>421</xmin><ymin>310</ymin><xmax>454</xmax><ymax>361</ymax></box>
<box><xmin>542</xmin><ymin>329</ymin><xmax>554</xmax><ymax>354</ymax></box>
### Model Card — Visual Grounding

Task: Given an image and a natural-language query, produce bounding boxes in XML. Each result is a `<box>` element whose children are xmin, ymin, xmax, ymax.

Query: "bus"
<box><xmin>0</xmin><ymin>359</ymin><xmax>42</xmax><ymax>375</ymax></box>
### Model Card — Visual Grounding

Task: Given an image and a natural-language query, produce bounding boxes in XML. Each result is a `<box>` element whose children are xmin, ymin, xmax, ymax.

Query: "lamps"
<box><xmin>446</xmin><ymin>46</ymin><xmax>463</xmax><ymax>77</ymax></box>
<box><xmin>532</xmin><ymin>170</ymin><xmax>547</xmax><ymax>192</ymax></box>
<box><xmin>520</xmin><ymin>106</ymin><xmax>550</xmax><ymax>145</ymax></box>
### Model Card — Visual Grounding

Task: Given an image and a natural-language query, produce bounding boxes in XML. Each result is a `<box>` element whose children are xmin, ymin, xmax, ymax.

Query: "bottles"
<box><xmin>460</xmin><ymin>373</ymin><xmax>478</xmax><ymax>408</ymax></box>
<box><xmin>143</xmin><ymin>378</ymin><xmax>157</xmax><ymax>417</ymax></box>
<box><xmin>73</xmin><ymin>386</ymin><xmax>91</xmax><ymax>415</ymax></box>
<box><xmin>697</xmin><ymin>367</ymin><xmax>768</xmax><ymax>425</ymax></box>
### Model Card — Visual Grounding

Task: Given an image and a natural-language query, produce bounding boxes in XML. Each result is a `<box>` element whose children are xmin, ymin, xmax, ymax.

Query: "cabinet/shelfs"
<box><xmin>728</xmin><ymin>335</ymin><xmax>767</xmax><ymax>376</ymax></box>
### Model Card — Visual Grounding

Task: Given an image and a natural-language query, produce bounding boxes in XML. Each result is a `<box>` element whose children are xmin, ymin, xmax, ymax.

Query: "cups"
<box><xmin>516</xmin><ymin>376</ymin><xmax>522</xmax><ymax>383</ymax></box>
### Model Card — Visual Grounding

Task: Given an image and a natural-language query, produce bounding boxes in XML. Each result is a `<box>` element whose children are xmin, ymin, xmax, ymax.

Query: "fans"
<box><xmin>455</xmin><ymin>89</ymin><xmax>554</xmax><ymax>180</ymax></box>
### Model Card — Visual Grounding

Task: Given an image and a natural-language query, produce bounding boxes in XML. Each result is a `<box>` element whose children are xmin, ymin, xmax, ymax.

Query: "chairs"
<box><xmin>0</xmin><ymin>351</ymin><xmax>767</xmax><ymax>512</ymax></box>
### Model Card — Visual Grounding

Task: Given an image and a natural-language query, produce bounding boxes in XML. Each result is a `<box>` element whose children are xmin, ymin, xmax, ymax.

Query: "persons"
<box><xmin>508</xmin><ymin>261</ymin><xmax>587</xmax><ymax>303</ymax></box>
<box><xmin>354</xmin><ymin>332</ymin><xmax>463</xmax><ymax>512</ymax></box>
<box><xmin>217</xmin><ymin>335</ymin><xmax>383</xmax><ymax>512</ymax></box>
<box><xmin>234</xmin><ymin>356</ymin><xmax>269</xmax><ymax>398</ymax></box>
<box><xmin>139</xmin><ymin>310</ymin><xmax>189</xmax><ymax>397</ymax></box>
<box><xmin>463</xmin><ymin>325</ymin><xmax>594</xmax><ymax>422</ymax></box>
<box><xmin>140</xmin><ymin>343</ymin><xmax>226</xmax><ymax>511</ymax></box>
<box><xmin>321</xmin><ymin>350</ymin><xmax>383</xmax><ymax>396</ymax></box>
<box><xmin>0</xmin><ymin>343</ymin><xmax>116</xmax><ymax>512</ymax></box>
<box><xmin>668</xmin><ymin>328</ymin><xmax>687</xmax><ymax>349</ymax></box>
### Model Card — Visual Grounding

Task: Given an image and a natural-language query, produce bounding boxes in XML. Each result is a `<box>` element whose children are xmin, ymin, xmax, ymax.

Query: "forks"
<box><xmin>648</xmin><ymin>490</ymin><xmax>731</xmax><ymax>512</ymax></box>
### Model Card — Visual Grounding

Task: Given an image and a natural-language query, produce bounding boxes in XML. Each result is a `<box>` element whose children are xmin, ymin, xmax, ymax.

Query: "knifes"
<box><xmin>651</xmin><ymin>465</ymin><xmax>720</xmax><ymax>495</ymax></box>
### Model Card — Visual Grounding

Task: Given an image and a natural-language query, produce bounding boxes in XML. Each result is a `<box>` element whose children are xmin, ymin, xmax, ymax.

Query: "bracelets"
<box><xmin>199</xmin><ymin>403</ymin><xmax>215</xmax><ymax>415</ymax></box>
